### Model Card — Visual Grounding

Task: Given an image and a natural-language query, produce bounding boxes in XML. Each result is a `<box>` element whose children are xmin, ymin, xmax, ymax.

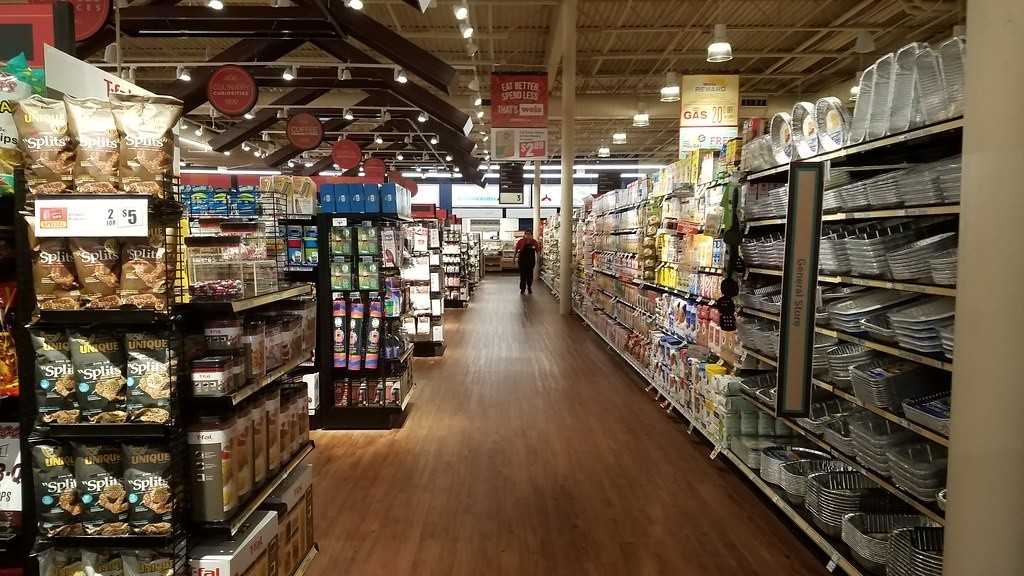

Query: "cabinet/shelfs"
<box><xmin>0</xmin><ymin>165</ymin><xmax>318</xmax><ymax>576</ymax></box>
<box><xmin>540</xmin><ymin>114</ymin><xmax>1024</xmax><ymax>575</ymax></box>
<box><xmin>276</xmin><ymin>213</ymin><xmax>417</xmax><ymax>432</ymax></box>
<box><xmin>403</xmin><ymin>194</ymin><xmax>518</xmax><ymax>358</ymax></box>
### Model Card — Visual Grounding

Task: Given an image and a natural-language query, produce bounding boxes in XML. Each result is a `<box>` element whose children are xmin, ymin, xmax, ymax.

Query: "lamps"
<box><xmin>177</xmin><ymin>65</ymin><xmax>192</xmax><ymax>82</ymax></box>
<box><xmin>121</xmin><ymin>65</ymin><xmax>135</xmax><ymax>85</ymax></box>
<box><xmin>853</xmin><ymin>29</ymin><xmax>875</xmax><ymax>53</ymax></box>
<box><xmin>706</xmin><ymin>0</ymin><xmax>733</xmax><ymax>62</ymax></box>
<box><xmin>178</xmin><ymin>107</ymin><xmax>459</xmax><ymax>176</ymax></box>
<box><xmin>283</xmin><ymin>64</ymin><xmax>299</xmax><ymax>80</ymax></box>
<box><xmin>660</xmin><ymin>50</ymin><xmax>681</xmax><ymax>103</ymax></box>
<box><xmin>113</xmin><ymin>0</ymin><xmax>129</xmax><ymax>9</ymax></box>
<box><xmin>204</xmin><ymin>0</ymin><xmax>224</xmax><ymax>10</ymax></box>
<box><xmin>337</xmin><ymin>64</ymin><xmax>352</xmax><ymax>80</ymax></box>
<box><xmin>105</xmin><ymin>43</ymin><xmax>125</xmax><ymax>64</ymax></box>
<box><xmin>393</xmin><ymin>66</ymin><xmax>408</xmax><ymax>83</ymax></box>
<box><xmin>270</xmin><ymin>0</ymin><xmax>290</xmax><ymax>8</ymax></box>
<box><xmin>452</xmin><ymin>0</ymin><xmax>491</xmax><ymax>166</ymax></box>
<box><xmin>598</xmin><ymin>145</ymin><xmax>611</xmax><ymax>157</ymax></box>
<box><xmin>344</xmin><ymin>0</ymin><xmax>363</xmax><ymax>11</ymax></box>
<box><xmin>632</xmin><ymin>101</ymin><xmax>650</xmax><ymax>126</ymax></box>
<box><xmin>613</xmin><ymin>124</ymin><xmax>627</xmax><ymax>145</ymax></box>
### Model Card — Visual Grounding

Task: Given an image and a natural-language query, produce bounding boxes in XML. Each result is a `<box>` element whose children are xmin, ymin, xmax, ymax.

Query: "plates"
<box><xmin>742</xmin><ymin>36</ymin><xmax>965</xmax><ymax>576</ymax></box>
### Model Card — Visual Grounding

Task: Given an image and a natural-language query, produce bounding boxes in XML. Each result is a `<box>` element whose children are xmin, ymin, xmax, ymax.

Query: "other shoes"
<box><xmin>520</xmin><ymin>289</ymin><xmax>525</xmax><ymax>293</ymax></box>
<box><xmin>527</xmin><ymin>287</ymin><xmax>532</xmax><ymax>294</ymax></box>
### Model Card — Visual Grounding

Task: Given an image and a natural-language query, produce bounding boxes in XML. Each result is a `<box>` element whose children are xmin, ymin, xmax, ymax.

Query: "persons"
<box><xmin>512</xmin><ymin>229</ymin><xmax>540</xmax><ymax>295</ymax></box>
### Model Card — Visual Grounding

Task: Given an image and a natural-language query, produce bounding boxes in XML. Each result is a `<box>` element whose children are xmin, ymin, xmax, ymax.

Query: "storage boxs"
<box><xmin>657</xmin><ymin>137</ymin><xmax>743</xmax><ymax>197</ymax></box>
<box><xmin>319</xmin><ymin>183</ymin><xmax>412</xmax><ymax>217</ymax></box>
<box><xmin>186</xmin><ymin>460</ymin><xmax>314</xmax><ymax>576</ymax></box>
<box><xmin>334</xmin><ymin>362</ymin><xmax>413</xmax><ymax>407</ymax></box>
